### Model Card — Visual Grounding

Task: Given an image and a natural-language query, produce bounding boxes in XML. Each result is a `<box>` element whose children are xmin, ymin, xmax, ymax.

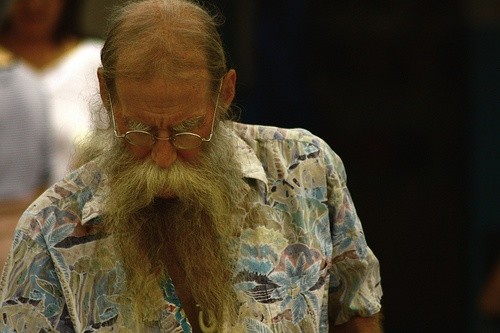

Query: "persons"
<box><xmin>0</xmin><ymin>0</ymin><xmax>383</xmax><ymax>333</ymax></box>
<box><xmin>0</xmin><ymin>0</ymin><xmax>105</xmax><ymax>280</ymax></box>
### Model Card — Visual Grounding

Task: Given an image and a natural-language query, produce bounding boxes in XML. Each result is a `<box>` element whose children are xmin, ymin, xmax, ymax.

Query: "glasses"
<box><xmin>107</xmin><ymin>74</ymin><xmax>225</xmax><ymax>150</ymax></box>
